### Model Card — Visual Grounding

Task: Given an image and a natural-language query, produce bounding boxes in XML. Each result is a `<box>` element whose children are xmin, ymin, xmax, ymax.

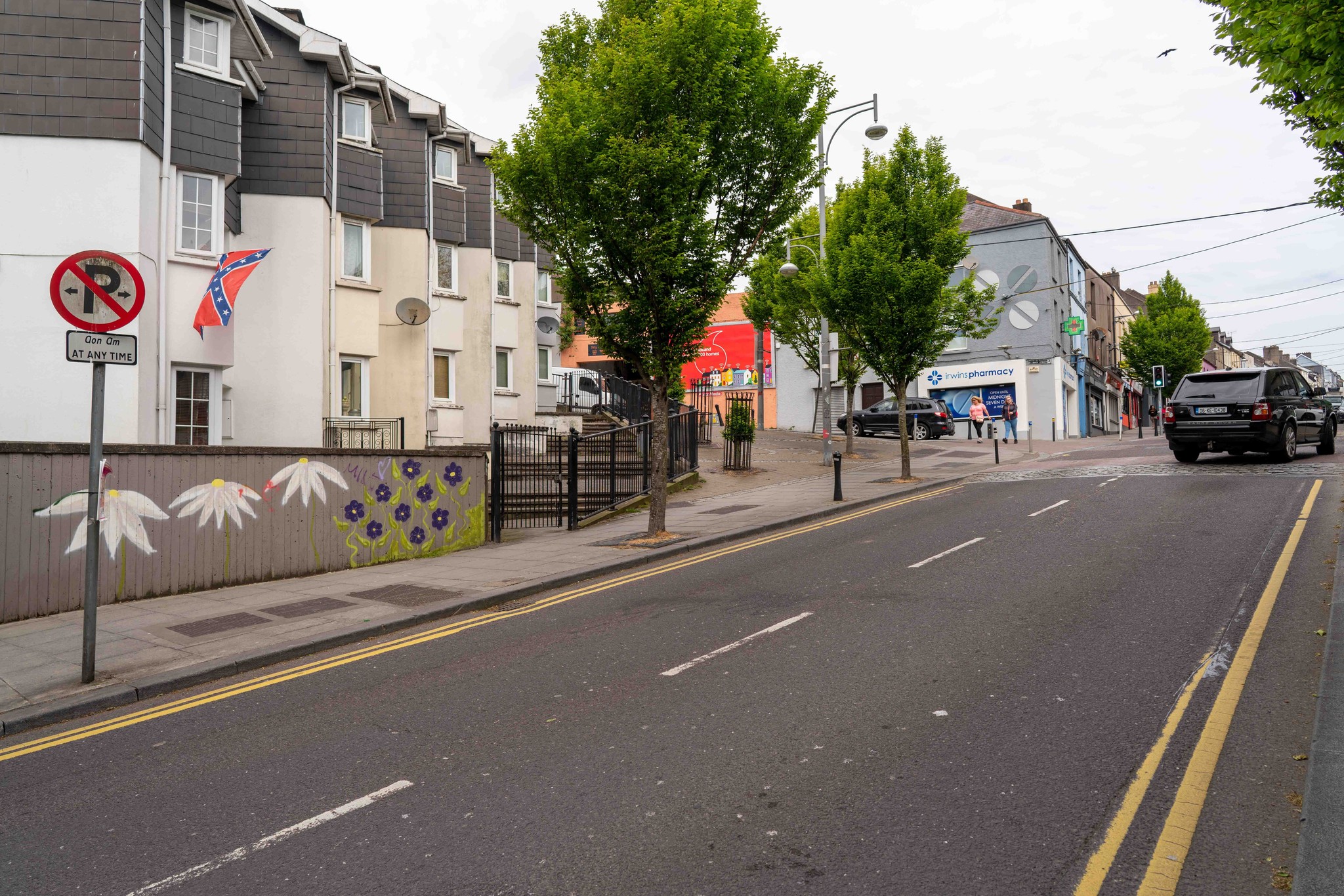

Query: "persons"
<box><xmin>1001</xmin><ymin>396</ymin><xmax>1018</xmax><ymax>444</ymax></box>
<box><xmin>1148</xmin><ymin>405</ymin><xmax>1157</xmax><ymax>429</ymax></box>
<box><xmin>969</xmin><ymin>396</ymin><xmax>992</xmax><ymax>443</ymax></box>
<box><xmin>1161</xmin><ymin>405</ymin><xmax>1166</xmax><ymax>429</ymax></box>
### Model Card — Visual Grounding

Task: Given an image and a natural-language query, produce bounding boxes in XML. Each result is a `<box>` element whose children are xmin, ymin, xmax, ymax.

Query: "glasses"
<box><xmin>972</xmin><ymin>399</ymin><xmax>977</xmax><ymax>402</ymax></box>
<box><xmin>1006</xmin><ymin>399</ymin><xmax>1011</xmax><ymax>401</ymax></box>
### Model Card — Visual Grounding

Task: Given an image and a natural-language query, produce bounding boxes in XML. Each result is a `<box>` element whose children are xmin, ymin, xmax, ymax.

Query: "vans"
<box><xmin>551</xmin><ymin>366</ymin><xmax>627</xmax><ymax>415</ymax></box>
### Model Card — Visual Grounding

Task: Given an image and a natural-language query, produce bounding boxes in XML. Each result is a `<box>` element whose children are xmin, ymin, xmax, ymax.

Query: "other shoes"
<box><xmin>1152</xmin><ymin>427</ymin><xmax>1154</xmax><ymax>429</ymax></box>
<box><xmin>1154</xmin><ymin>427</ymin><xmax>1155</xmax><ymax>429</ymax></box>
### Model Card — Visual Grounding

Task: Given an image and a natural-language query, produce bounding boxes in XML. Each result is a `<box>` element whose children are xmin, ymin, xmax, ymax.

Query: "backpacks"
<box><xmin>1004</xmin><ymin>403</ymin><xmax>1018</xmax><ymax>418</ymax></box>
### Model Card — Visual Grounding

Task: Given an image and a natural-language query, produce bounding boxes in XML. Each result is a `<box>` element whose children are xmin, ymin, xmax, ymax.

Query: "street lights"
<box><xmin>779</xmin><ymin>93</ymin><xmax>890</xmax><ymax>467</ymax></box>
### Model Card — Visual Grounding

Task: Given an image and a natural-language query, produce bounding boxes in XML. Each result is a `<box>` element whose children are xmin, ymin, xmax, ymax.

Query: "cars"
<box><xmin>1336</xmin><ymin>399</ymin><xmax>1344</xmax><ymax>423</ymax></box>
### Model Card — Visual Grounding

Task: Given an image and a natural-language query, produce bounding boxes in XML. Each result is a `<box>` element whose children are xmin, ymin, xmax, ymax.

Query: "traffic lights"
<box><xmin>1164</xmin><ymin>369</ymin><xmax>1172</xmax><ymax>387</ymax></box>
<box><xmin>1152</xmin><ymin>365</ymin><xmax>1164</xmax><ymax>388</ymax></box>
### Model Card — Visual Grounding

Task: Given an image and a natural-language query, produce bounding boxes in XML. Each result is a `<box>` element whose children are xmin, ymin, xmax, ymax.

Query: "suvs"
<box><xmin>837</xmin><ymin>396</ymin><xmax>956</xmax><ymax>440</ymax></box>
<box><xmin>1322</xmin><ymin>395</ymin><xmax>1344</xmax><ymax>417</ymax></box>
<box><xmin>1152</xmin><ymin>364</ymin><xmax>1337</xmax><ymax>463</ymax></box>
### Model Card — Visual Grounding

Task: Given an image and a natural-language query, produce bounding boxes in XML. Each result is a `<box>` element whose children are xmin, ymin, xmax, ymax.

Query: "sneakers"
<box><xmin>1014</xmin><ymin>439</ymin><xmax>1018</xmax><ymax>443</ymax></box>
<box><xmin>977</xmin><ymin>440</ymin><xmax>983</xmax><ymax>443</ymax></box>
<box><xmin>1003</xmin><ymin>438</ymin><xmax>1008</xmax><ymax>444</ymax></box>
<box><xmin>977</xmin><ymin>439</ymin><xmax>982</xmax><ymax>443</ymax></box>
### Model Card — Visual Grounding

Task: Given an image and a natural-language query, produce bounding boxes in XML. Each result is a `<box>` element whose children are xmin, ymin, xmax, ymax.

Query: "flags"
<box><xmin>193</xmin><ymin>248</ymin><xmax>273</xmax><ymax>342</ymax></box>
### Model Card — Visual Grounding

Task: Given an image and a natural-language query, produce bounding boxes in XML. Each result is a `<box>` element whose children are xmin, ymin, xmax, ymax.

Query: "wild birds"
<box><xmin>1156</xmin><ymin>48</ymin><xmax>1178</xmax><ymax>58</ymax></box>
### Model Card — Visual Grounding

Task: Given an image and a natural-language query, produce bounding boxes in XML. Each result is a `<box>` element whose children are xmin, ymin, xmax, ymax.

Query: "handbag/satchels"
<box><xmin>976</xmin><ymin>416</ymin><xmax>983</xmax><ymax>423</ymax></box>
